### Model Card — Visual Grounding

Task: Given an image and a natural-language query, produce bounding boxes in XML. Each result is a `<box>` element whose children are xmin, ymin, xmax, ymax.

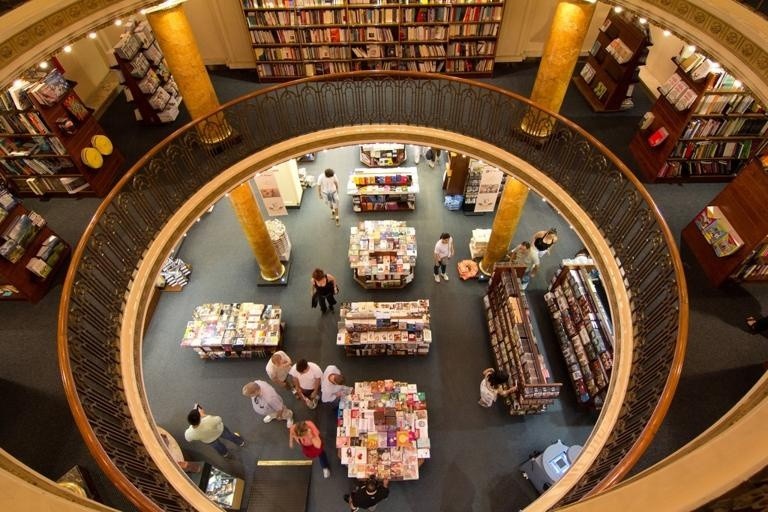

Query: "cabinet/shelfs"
<box><xmin>107</xmin><ymin>22</ymin><xmax>183</xmax><ymax>127</ymax></box>
<box><xmin>628</xmin><ymin>50</ymin><xmax>768</xmax><ymax>288</ymax></box>
<box><xmin>566</xmin><ymin>4</ymin><xmax>655</xmax><ymax>116</ymax></box>
<box><xmin>238</xmin><ymin>0</ymin><xmax>508</xmax><ymax>82</ymax></box>
<box><xmin>0</xmin><ymin>185</ymin><xmax>69</xmax><ymax>304</ymax></box>
<box><xmin>1</xmin><ymin>63</ymin><xmax>124</xmax><ymax>198</ymax></box>
<box><xmin>483</xmin><ymin>258</ymin><xmax>615</xmax><ymax>426</ymax></box>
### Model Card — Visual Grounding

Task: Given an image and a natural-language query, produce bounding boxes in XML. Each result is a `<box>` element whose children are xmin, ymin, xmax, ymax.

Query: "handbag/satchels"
<box><xmin>301</xmin><ymin>439</ymin><xmax>325</xmax><ymax>458</ymax></box>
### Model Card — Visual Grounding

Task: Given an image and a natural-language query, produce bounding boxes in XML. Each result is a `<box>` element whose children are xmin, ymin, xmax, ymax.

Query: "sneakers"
<box><xmin>322</xmin><ymin>468</ymin><xmax>330</xmax><ymax>478</ymax></box>
<box><xmin>331</xmin><ymin>210</ymin><xmax>340</xmax><ymax>227</ymax></box>
<box><xmin>263</xmin><ymin>415</ymin><xmax>276</xmax><ymax>424</ymax></box>
<box><xmin>291</xmin><ymin>389</ymin><xmax>300</xmax><ymax>400</ymax></box>
<box><xmin>222</xmin><ymin>432</ymin><xmax>245</xmax><ymax>460</ymax></box>
<box><xmin>441</xmin><ymin>272</ymin><xmax>450</xmax><ymax>282</ymax></box>
<box><xmin>434</xmin><ymin>273</ymin><xmax>441</xmax><ymax>283</ymax></box>
<box><xmin>285</xmin><ymin>410</ymin><xmax>294</xmax><ymax>428</ymax></box>
